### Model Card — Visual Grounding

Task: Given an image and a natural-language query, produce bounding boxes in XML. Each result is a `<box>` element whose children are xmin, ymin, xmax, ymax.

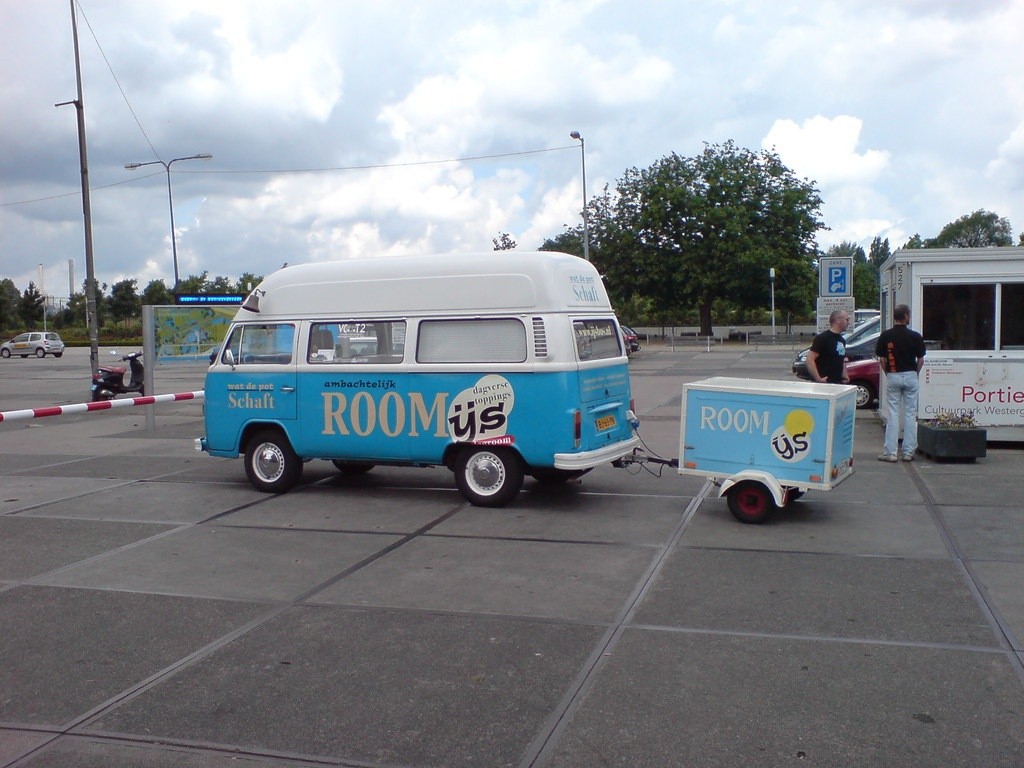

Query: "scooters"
<box><xmin>88</xmin><ymin>347</ymin><xmax>145</xmax><ymax>402</ymax></box>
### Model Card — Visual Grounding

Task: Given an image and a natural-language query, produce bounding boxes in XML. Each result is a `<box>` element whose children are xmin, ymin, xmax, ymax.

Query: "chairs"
<box><xmin>312</xmin><ymin>329</ymin><xmax>334</xmax><ymax>350</ymax></box>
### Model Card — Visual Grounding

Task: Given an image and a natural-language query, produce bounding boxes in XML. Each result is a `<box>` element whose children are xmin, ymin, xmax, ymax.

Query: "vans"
<box><xmin>191</xmin><ymin>251</ymin><xmax>643</xmax><ymax>509</ymax></box>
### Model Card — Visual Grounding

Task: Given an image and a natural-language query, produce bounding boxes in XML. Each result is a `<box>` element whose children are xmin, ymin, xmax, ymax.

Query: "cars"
<box><xmin>0</xmin><ymin>331</ymin><xmax>65</xmax><ymax>358</ymax></box>
<box><xmin>845</xmin><ymin>332</ymin><xmax>880</xmax><ymax>362</ymax></box>
<box><xmin>790</xmin><ymin>313</ymin><xmax>881</xmax><ymax>381</ymax></box>
<box><xmin>620</xmin><ymin>325</ymin><xmax>641</xmax><ymax>352</ymax></box>
<box><xmin>620</xmin><ymin>328</ymin><xmax>632</xmax><ymax>357</ymax></box>
<box><xmin>842</xmin><ymin>356</ymin><xmax>882</xmax><ymax>410</ymax></box>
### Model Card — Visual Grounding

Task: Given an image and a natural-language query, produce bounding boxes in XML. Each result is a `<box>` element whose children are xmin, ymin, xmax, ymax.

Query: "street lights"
<box><xmin>769</xmin><ymin>267</ymin><xmax>776</xmax><ymax>343</ymax></box>
<box><xmin>569</xmin><ymin>130</ymin><xmax>595</xmax><ymax>260</ymax></box>
<box><xmin>123</xmin><ymin>154</ymin><xmax>215</xmax><ymax>289</ymax></box>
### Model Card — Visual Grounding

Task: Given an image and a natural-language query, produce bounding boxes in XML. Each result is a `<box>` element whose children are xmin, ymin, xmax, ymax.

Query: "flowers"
<box><xmin>924</xmin><ymin>410</ymin><xmax>978</xmax><ymax>429</ymax></box>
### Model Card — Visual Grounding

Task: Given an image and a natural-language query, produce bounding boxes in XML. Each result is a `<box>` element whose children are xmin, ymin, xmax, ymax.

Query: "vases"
<box><xmin>917</xmin><ymin>422</ymin><xmax>987</xmax><ymax>462</ymax></box>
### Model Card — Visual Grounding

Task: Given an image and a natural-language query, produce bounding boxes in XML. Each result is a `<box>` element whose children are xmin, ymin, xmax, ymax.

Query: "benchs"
<box><xmin>667</xmin><ymin>336</ymin><xmax>717</xmax><ymax>352</ymax></box>
<box><xmin>749</xmin><ymin>334</ymin><xmax>800</xmax><ymax>351</ymax></box>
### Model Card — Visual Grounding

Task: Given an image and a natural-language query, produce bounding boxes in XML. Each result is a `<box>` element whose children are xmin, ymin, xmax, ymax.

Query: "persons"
<box><xmin>875</xmin><ymin>304</ymin><xmax>926</xmax><ymax>463</ymax></box>
<box><xmin>804</xmin><ymin>310</ymin><xmax>850</xmax><ymax>385</ymax></box>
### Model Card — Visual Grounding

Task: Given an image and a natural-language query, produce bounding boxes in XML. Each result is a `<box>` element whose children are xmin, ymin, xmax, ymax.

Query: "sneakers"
<box><xmin>878</xmin><ymin>454</ymin><xmax>898</xmax><ymax>462</ymax></box>
<box><xmin>902</xmin><ymin>454</ymin><xmax>912</xmax><ymax>461</ymax></box>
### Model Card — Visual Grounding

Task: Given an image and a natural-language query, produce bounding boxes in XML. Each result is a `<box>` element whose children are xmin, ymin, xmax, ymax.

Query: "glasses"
<box><xmin>838</xmin><ymin>318</ymin><xmax>850</xmax><ymax>323</ymax></box>
